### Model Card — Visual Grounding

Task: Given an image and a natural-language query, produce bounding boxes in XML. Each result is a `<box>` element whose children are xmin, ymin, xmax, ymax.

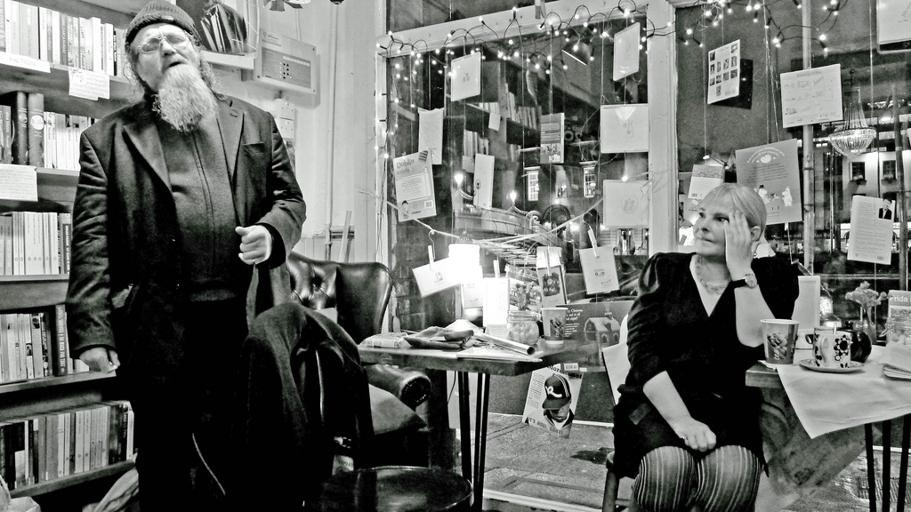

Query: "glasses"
<box><xmin>133</xmin><ymin>35</ymin><xmax>191</xmax><ymax>69</ymax></box>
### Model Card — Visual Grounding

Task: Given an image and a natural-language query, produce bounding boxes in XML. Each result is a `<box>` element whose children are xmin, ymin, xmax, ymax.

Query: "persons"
<box><xmin>611</xmin><ymin>181</ymin><xmax>800</xmax><ymax>511</ymax></box>
<box><xmin>66</xmin><ymin>0</ymin><xmax>306</xmax><ymax>511</ymax></box>
<box><xmin>878</xmin><ymin>197</ymin><xmax>892</xmax><ymax>220</ymax></box>
<box><xmin>524</xmin><ymin>374</ymin><xmax>574</xmax><ymax>439</ymax></box>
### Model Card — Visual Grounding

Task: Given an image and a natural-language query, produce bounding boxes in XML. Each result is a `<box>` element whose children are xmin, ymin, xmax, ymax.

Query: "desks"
<box><xmin>746</xmin><ymin>344</ymin><xmax>911</xmax><ymax>512</ymax></box>
<box><xmin>357</xmin><ymin>332</ymin><xmax>599</xmax><ymax>512</ymax></box>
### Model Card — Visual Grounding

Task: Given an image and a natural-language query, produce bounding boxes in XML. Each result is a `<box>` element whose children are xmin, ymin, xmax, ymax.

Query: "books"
<box><xmin>0</xmin><ymin>400</ymin><xmax>135</xmax><ymax>490</ymax></box>
<box><xmin>877</xmin><ymin>341</ymin><xmax>910</xmax><ymax>382</ymax></box>
<box><xmin>0</xmin><ymin>212</ymin><xmax>72</xmax><ymax>277</ymax></box>
<box><xmin>0</xmin><ymin>1</ymin><xmax>141</xmax><ymax>83</ymax></box>
<box><xmin>0</xmin><ymin>91</ymin><xmax>100</xmax><ymax>170</ymax></box>
<box><xmin>0</xmin><ymin>305</ymin><xmax>88</xmax><ymax>384</ymax></box>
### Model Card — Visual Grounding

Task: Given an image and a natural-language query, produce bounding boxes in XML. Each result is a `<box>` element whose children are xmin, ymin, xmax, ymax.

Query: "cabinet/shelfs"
<box><xmin>1</xmin><ymin>0</ymin><xmax>175</xmax><ymax>498</ymax></box>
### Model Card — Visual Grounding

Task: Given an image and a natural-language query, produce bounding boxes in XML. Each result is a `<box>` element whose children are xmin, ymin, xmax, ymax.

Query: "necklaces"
<box><xmin>692</xmin><ymin>253</ymin><xmax>730</xmax><ymax>295</ymax></box>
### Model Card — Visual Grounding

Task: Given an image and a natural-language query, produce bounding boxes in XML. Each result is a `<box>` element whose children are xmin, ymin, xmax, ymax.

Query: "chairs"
<box><xmin>601</xmin><ymin>342</ymin><xmax>631</xmax><ymax>512</ymax></box>
<box><xmin>285</xmin><ymin>251</ymin><xmax>474</xmax><ymax>512</ymax></box>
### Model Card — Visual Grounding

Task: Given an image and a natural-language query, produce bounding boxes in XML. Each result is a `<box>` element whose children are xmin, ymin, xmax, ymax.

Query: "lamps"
<box><xmin>828</xmin><ymin>68</ymin><xmax>877</xmax><ymax>157</ymax></box>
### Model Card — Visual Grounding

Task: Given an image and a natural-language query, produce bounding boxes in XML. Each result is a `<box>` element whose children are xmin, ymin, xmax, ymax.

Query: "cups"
<box><xmin>813</xmin><ymin>331</ymin><xmax>852</xmax><ymax>369</ymax></box>
<box><xmin>540</xmin><ymin>307</ymin><xmax>568</xmax><ymax>345</ymax></box>
<box><xmin>760</xmin><ymin>318</ymin><xmax>800</xmax><ymax>365</ymax></box>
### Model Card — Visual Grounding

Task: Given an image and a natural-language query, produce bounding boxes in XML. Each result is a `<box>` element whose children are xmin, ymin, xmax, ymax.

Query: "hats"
<box><xmin>126</xmin><ymin>0</ymin><xmax>195</xmax><ymax>43</ymax></box>
<box><xmin>542</xmin><ymin>375</ymin><xmax>570</xmax><ymax>411</ymax></box>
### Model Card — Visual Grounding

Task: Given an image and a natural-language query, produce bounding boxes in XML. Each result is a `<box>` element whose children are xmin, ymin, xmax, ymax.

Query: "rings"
<box><xmin>680</xmin><ymin>435</ymin><xmax>688</xmax><ymax>442</ymax></box>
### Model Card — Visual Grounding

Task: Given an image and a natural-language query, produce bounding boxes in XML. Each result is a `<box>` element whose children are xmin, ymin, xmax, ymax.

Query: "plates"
<box><xmin>801</xmin><ymin>360</ymin><xmax>864</xmax><ymax>372</ymax></box>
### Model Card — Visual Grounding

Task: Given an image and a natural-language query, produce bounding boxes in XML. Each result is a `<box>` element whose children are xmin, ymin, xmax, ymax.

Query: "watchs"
<box><xmin>732</xmin><ymin>273</ymin><xmax>759</xmax><ymax>291</ymax></box>
